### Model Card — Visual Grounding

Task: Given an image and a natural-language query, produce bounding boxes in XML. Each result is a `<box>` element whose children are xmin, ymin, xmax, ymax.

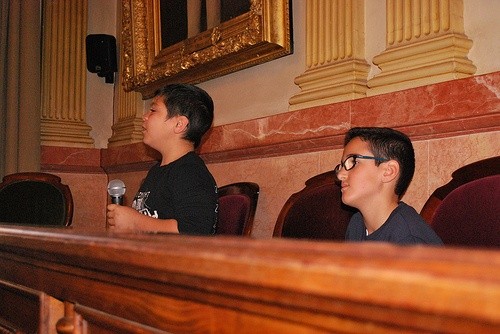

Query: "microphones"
<box><xmin>107</xmin><ymin>180</ymin><xmax>127</xmax><ymax>206</ymax></box>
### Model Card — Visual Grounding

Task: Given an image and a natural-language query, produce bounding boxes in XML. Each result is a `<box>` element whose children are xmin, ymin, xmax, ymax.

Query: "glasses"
<box><xmin>333</xmin><ymin>154</ymin><xmax>386</xmax><ymax>175</ymax></box>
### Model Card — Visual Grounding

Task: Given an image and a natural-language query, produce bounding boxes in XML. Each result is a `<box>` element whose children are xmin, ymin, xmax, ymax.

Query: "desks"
<box><xmin>0</xmin><ymin>225</ymin><xmax>500</xmax><ymax>334</ymax></box>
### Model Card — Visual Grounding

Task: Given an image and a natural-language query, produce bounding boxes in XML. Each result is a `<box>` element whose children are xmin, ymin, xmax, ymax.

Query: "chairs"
<box><xmin>419</xmin><ymin>156</ymin><xmax>500</xmax><ymax>248</ymax></box>
<box><xmin>217</xmin><ymin>182</ymin><xmax>260</xmax><ymax>237</ymax></box>
<box><xmin>0</xmin><ymin>172</ymin><xmax>74</xmax><ymax>227</ymax></box>
<box><xmin>272</xmin><ymin>170</ymin><xmax>359</xmax><ymax>241</ymax></box>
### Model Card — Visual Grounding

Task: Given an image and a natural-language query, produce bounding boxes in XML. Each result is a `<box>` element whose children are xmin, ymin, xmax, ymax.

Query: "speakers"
<box><xmin>85</xmin><ymin>34</ymin><xmax>116</xmax><ymax>75</ymax></box>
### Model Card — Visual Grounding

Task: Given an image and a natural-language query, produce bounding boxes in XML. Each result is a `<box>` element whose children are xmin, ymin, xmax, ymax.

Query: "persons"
<box><xmin>107</xmin><ymin>83</ymin><xmax>218</xmax><ymax>236</ymax></box>
<box><xmin>334</xmin><ymin>127</ymin><xmax>443</xmax><ymax>245</ymax></box>
